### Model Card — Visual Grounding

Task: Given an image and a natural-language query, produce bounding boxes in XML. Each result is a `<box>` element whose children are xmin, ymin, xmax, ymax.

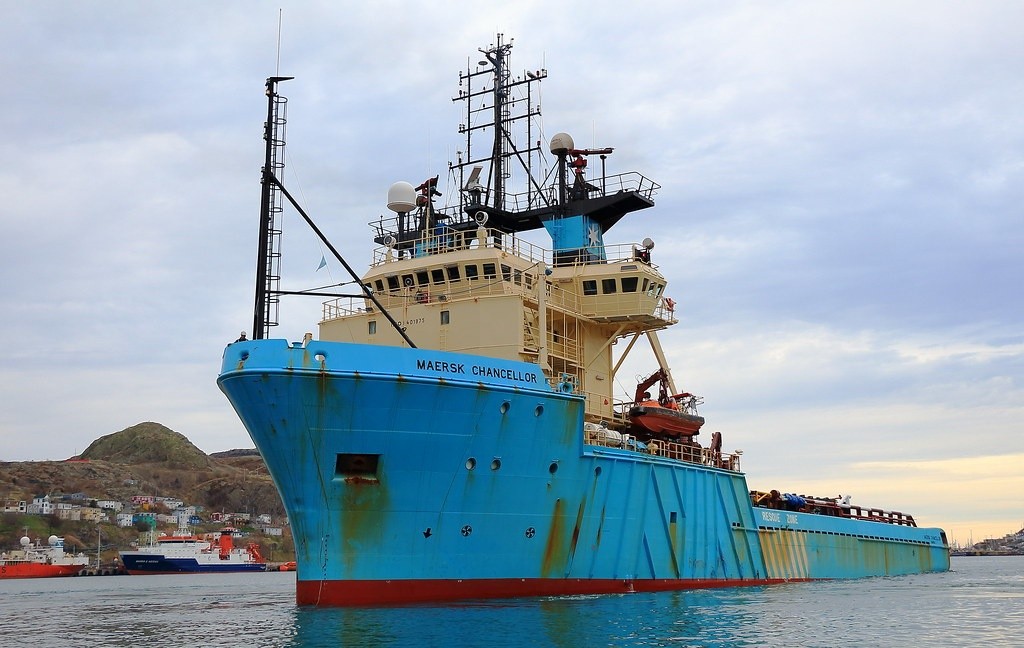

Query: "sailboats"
<box><xmin>217</xmin><ymin>8</ymin><xmax>951</xmax><ymax>606</ymax></box>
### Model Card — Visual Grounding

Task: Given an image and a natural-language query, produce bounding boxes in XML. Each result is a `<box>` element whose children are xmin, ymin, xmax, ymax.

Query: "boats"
<box><xmin>0</xmin><ymin>535</ymin><xmax>85</xmax><ymax>578</ymax></box>
<box><xmin>119</xmin><ymin>525</ymin><xmax>267</xmax><ymax>573</ymax></box>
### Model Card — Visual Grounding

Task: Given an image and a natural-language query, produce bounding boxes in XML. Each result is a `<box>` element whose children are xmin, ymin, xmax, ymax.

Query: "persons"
<box><xmin>234</xmin><ymin>331</ymin><xmax>249</xmax><ymax>343</ymax></box>
